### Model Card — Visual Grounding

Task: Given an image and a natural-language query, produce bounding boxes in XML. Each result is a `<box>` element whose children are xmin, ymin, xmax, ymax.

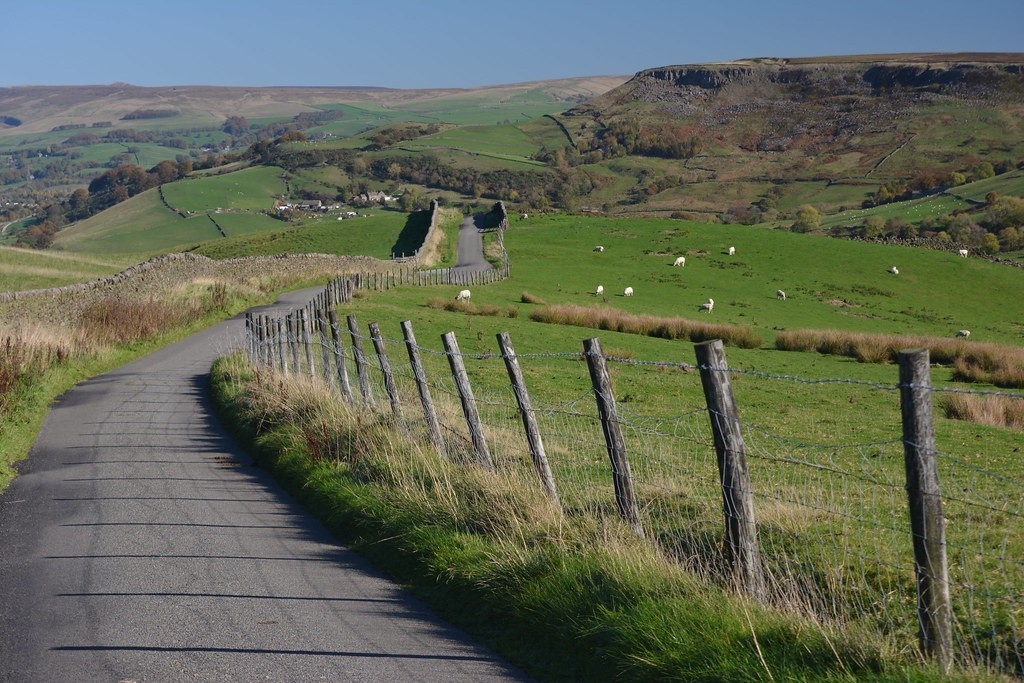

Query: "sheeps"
<box><xmin>456</xmin><ymin>290</ymin><xmax>470</xmax><ymax>303</ymax></box>
<box><xmin>520</xmin><ymin>214</ymin><xmax>527</xmax><ymax>221</ymax></box>
<box><xmin>728</xmin><ymin>246</ymin><xmax>736</xmax><ymax>256</ymax></box>
<box><xmin>955</xmin><ymin>330</ymin><xmax>970</xmax><ymax>339</ymax></box>
<box><xmin>595</xmin><ymin>246</ymin><xmax>603</xmax><ymax>253</ymax></box>
<box><xmin>956</xmin><ymin>249</ymin><xmax>968</xmax><ymax>257</ymax></box>
<box><xmin>702</xmin><ymin>299</ymin><xmax>714</xmax><ymax>314</ymax></box>
<box><xmin>673</xmin><ymin>257</ymin><xmax>685</xmax><ymax>267</ymax></box>
<box><xmin>595</xmin><ymin>285</ymin><xmax>603</xmax><ymax>296</ymax></box>
<box><xmin>891</xmin><ymin>266</ymin><xmax>899</xmax><ymax>275</ymax></box>
<box><xmin>624</xmin><ymin>287</ymin><xmax>633</xmax><ymax>297</ymax></box>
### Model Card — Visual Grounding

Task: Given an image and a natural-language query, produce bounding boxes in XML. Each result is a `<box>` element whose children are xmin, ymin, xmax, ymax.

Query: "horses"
<box><xmin>777</xmin><ymin>290</ymin><xmax>786</xmax><ymax>301</ymax></box>
